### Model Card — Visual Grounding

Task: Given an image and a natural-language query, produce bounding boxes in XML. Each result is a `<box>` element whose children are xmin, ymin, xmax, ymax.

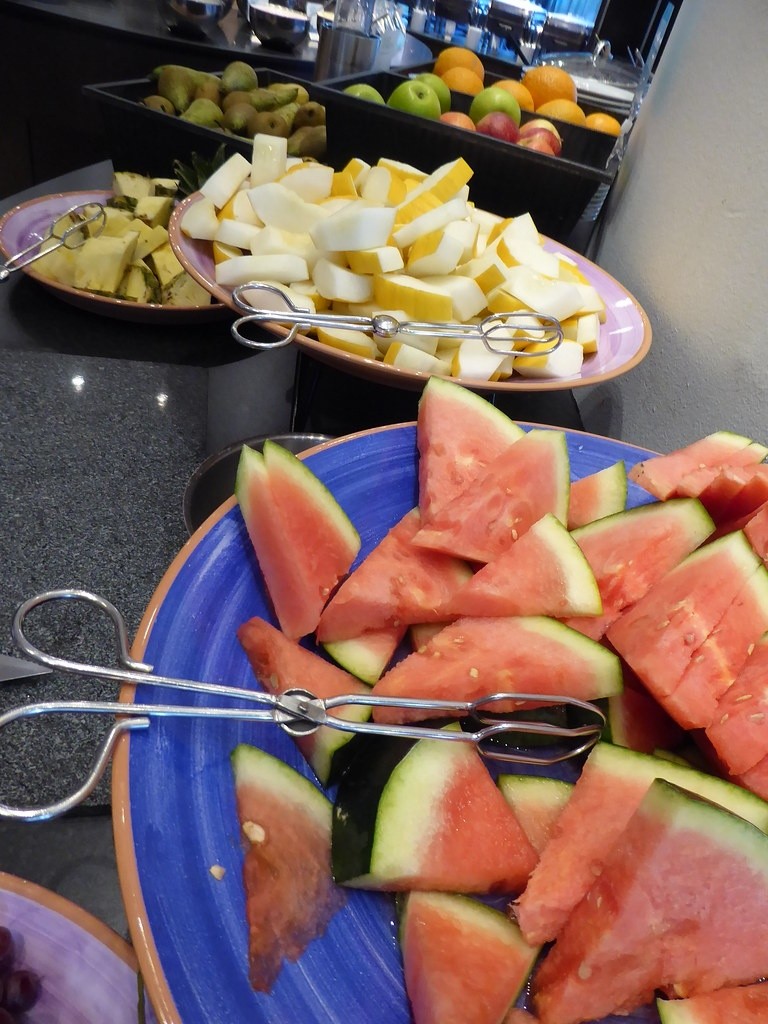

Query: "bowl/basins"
<box><xmin>249</xmin><ymin>3</ymin><xmax>311</xmax><ymax>50</ymax></box>
<box><xmin>169</xmin><ymin>0</ymin><xmax>234</xmax><ymax>23</ymax></box>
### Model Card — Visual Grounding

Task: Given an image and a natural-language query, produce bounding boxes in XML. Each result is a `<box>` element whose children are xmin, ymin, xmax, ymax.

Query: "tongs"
<box><xmin>0</xmin><ymin>202</ymin><xmax>106</xmax><ymax>280</ymax></box>
<box><xmin>232</xmin><ymin>284</ymin><xmax>564</xmax><ymax>356</ymax></box>
<box><xmin>2</xmin><ymin>587</ymin><xmax>607</xmax><ymax>823</ymax></box>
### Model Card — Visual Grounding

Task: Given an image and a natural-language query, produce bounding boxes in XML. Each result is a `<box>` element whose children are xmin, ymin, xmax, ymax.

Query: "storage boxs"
<box><xmin>83</xmin><ymin>59</ymin><xmax>633</xmax><ymax>244</ymax></box>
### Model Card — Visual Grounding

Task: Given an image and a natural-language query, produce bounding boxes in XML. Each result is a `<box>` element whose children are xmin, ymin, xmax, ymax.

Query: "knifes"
<box><xmin>0</xmin><ymin>654</ymin><xmax>52</xmax><ymax>682</ymax></box>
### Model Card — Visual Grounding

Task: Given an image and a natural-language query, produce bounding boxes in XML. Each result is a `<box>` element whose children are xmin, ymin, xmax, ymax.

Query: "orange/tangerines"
<box><xmin>436</xmin><ymin>49</ymin><xmax>619</xmax><ymax>134</ymax></box>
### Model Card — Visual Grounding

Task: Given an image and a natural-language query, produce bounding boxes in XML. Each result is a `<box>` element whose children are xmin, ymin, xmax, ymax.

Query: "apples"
<box><xmin>340</xmin><ymin>72</ymin><xmax>562</xmax><ymax>158</ymax></box>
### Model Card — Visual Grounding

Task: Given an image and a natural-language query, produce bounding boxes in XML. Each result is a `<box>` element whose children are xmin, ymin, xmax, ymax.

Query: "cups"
<box><xmin>182</xmin><ymin>436</ymin><xmax>330</xmax><ymax>532</ymax></box>
<box><xmin>313</xmin><ymin>25</ymin><xmax>382</xmax><ymax>84</ymax></box>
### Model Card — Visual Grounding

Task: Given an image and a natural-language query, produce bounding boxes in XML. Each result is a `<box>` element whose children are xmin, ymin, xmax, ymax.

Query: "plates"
<box><xmin>0</xmin><ymin>872</ymin><xmax>161</xmax><ymax>1024</ymax></box>
<box><xmin>0</xmin><ymin>192</ymin><xmax>225</xmax><ymax>310</ymax></box>
<box><xmin>168</xmin><ymin>180</ymin><xmax>652</xmax><ymax>390</ymax></box>
<box><xmin>111</xmin><ymin>425</ymin><xmax>768</xmax><ymax>1024</ymax></box>
<box><xmin>521</xmin><ymin>65</ymin><xmax>642</xmax><ymax>110</ymax></box>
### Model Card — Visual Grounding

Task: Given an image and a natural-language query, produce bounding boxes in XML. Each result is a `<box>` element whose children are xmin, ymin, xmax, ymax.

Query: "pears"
<box><xmin>137</xmin><ymin>61</ymin><xmax>326</xmax><ymax>162</ymax></box>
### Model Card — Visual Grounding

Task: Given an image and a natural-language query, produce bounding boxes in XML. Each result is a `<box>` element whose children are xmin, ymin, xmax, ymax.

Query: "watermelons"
<box><xmin>234</xmin><ymin>377</ymin><xmax>768</xmax><ymax>1024</ymax></box>
